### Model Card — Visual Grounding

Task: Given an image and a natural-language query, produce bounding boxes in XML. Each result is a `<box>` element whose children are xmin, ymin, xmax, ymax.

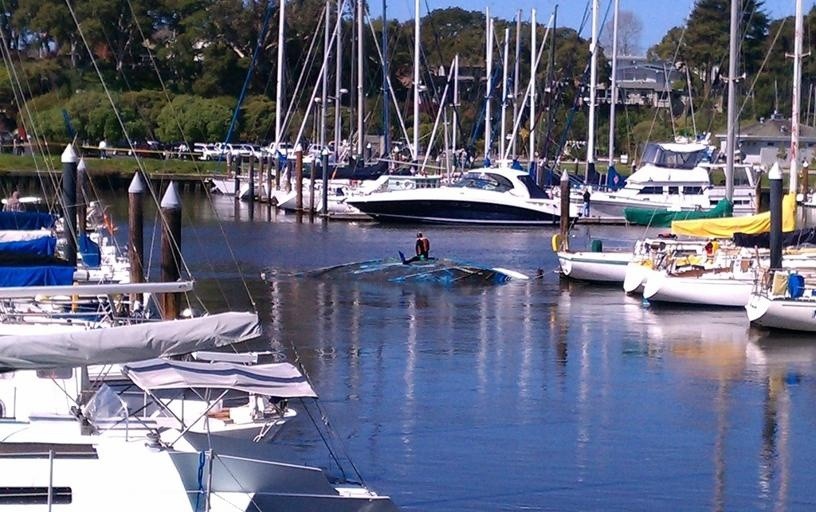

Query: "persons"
<box><xmin>15</xmin><ymin>135</ymin><xmax>25</xmax><ymax>156</ymax></box>
<box><xmin>582</xmin><ymin>187</ymin><xmax>591</xmax><ymax>217</ymax></box>
<box><xmin>13</xmin><ymin>133</ymin><xmax>18</xmax><ymax>154</ymax></box>
<box><xmin>704</xmin><ymin>238</ymin><xmax>714</xmax><ymax>264</ymax></box>
<box><xmin>656</xmin><ymin>241</ymin><xmax>668</xmax><ymax>269</ymax></box>
<box><xmin>3</xmin><ymin>192</ymin><xmax>27</xmax><ymax>212</ymax></box>
<box><xmin>97</xmin><ymin>136</ymin><xmax>107</xmax><ymax>160</ymax></box>
<box><xmin>700</xmin><ymin>130</ymin><xmax>707</xmax><ymax>141</ymax></box>
<box><xmin>712</xmin><ymin>238</ymin><xmax>719</xmax><ymax>261</ymax></box>
<box><xmin>404</xmin><ymin>232</ymin><xmax>431</xmax><ymax>264</ymax></box>
<box><xmin>556</xmin><ymin>156</ymin><xmax>562</xmax><ymax>174</ymax></box>
